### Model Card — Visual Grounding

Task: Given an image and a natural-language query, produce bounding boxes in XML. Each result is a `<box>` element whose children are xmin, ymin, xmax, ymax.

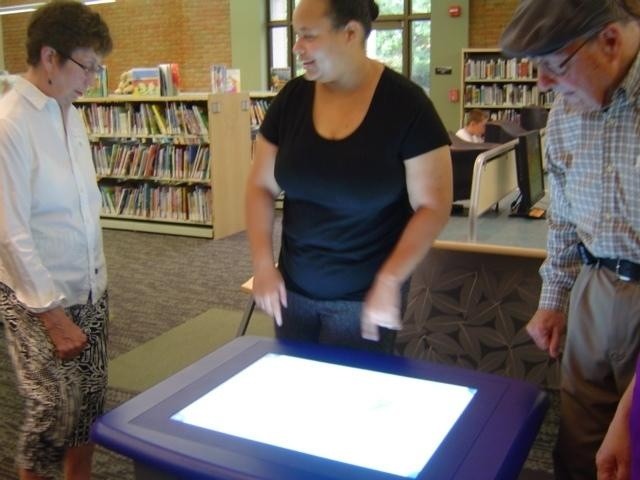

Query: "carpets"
<box><xmin>106</xmin><ymin>307</ymin><xmax>275</xmax><ymax>394</ymax></box>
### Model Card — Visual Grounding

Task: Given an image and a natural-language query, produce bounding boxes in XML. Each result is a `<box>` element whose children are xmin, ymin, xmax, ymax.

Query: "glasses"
<box><xmin>58</xmin><ymin>50</ymin><xmax>105</xmax><ymax>78</ymax></box>
<box><xmin>542</xmin><ymin>30</ymin><xmax>599</xmax><ymax>77</ymax></box>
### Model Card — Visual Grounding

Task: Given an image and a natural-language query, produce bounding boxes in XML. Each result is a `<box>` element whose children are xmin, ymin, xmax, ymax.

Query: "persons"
<box><xmin>499</xmin><ymin>0</ymin><xmax>639</xmax><ymax>480</ymax></box>
<box><xmin>244</xmin><ymin>0</ymin><xmax>455</xmax><ymax>352</ymax></box>
<box><xmin>0</xmin><ymin>0</ymin><xmax>112</xmax><ymax>479</ymax></box>
<box><xmin>456</xmin><ymin>109</ymin><xmax>490</xmax><ymax>145</ymax></box>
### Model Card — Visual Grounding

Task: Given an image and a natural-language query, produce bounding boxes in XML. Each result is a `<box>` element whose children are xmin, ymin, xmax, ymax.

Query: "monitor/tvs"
<box><xmin>521</xmin><ymin>104</ymin><xmax>548</xmax><ymax>130</ymax></box>
<box><xmin>484</xmin><ymin>120</ymin><xmax>519</xmax><ymax>142</ymax></box>
<box><xmin>447</xmin><ymin>131</ymin><xmax>498</xmax><ymax>215</ymax></box>
<box><xmin>91</xmin><ymin>333</ymin><xmax>546</xmax><ymax>480</ymax></box>
<box><xmin>508</xmin><ymin>130</ymin><xmax>547</xmax><ymax>218</ymax></box>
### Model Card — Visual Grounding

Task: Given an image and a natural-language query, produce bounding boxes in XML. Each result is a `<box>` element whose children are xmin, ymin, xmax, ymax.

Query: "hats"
<box><xmin>496</xmin><ymin>0</ymin><xmax>625</xmax><ymax>60</ymax></box>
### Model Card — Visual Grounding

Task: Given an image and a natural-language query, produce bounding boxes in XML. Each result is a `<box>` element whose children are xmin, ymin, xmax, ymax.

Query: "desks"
<box><xmin>391</xmin><ymin>165</ymin><xmax>563</xmax><ymax>392</ymax></box>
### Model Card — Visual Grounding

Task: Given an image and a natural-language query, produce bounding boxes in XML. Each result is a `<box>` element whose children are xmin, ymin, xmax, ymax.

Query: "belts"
<box><xmin>599</xmin><ymin>258</ymin><xmax>639</xmax><ymax>283</ymax></box>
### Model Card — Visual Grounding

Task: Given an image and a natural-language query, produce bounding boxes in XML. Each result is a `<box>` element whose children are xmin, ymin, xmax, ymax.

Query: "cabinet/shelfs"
<box><xmin>71</xmin><ymin>91</ymin><xmax>283</xmax><ymax>240</ymax></box>
<box><xmin>460</xmin><ymin>47</ymin><xmax>558</xmax><ymax>138</ymax></box>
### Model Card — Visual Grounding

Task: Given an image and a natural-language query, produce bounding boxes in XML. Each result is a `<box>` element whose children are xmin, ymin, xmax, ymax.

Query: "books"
<box><xmin>249</xmin><ymin>96</ymin><xmax>277</xmax><ymax>143</ymax></box>
<box><xmin>70</xmin><ymin>62</ymin><xmax>242</xmax><ymax>225</ymax></box>
<box><xmin>462</xmin><ymin>52</ymin><xmax>554</xmax><ymax>129</ymax></box>
